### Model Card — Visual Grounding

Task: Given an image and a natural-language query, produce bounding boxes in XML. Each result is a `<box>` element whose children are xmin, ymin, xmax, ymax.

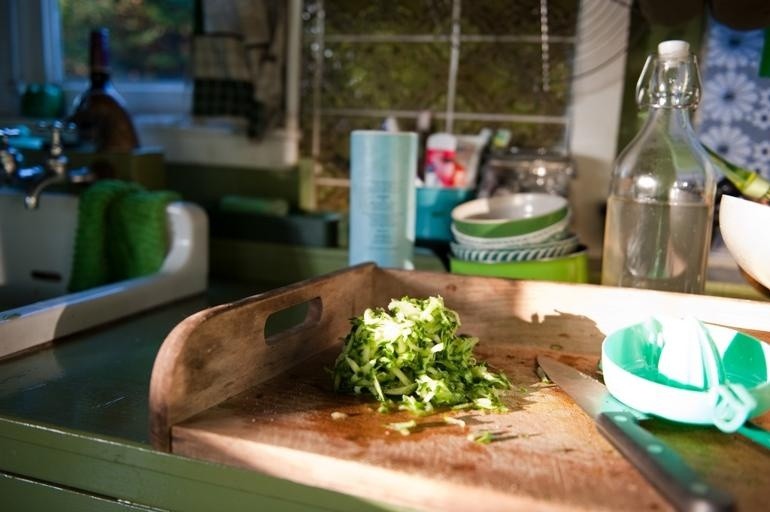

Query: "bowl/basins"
<box><xmin>447</xmin><ymin>191</ymin><xmax>584</xmax><ymax>275</ymax></box>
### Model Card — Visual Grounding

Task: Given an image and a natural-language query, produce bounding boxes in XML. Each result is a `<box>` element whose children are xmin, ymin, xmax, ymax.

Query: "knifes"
<box><xmin>533</xmin><ymin>352</ymin><xmax>735</xmax><ymax>512</ymax></box>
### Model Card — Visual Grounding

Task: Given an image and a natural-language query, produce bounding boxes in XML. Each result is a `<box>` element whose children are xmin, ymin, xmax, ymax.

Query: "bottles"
<box><xmin>71</xmin><ymin>23</ymin><xmax>140</xmax><ymax>150</ymax></box>
<box><xmin>603</xmin><ymin>40</ymin><xmax>716</xmax><ymax>290</ymax></box>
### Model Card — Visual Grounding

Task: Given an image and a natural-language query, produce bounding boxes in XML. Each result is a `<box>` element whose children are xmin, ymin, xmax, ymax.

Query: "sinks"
<box><xmin>0</xmin><ymin>188</ymin><xmax>210</xmax><ymax>360</ymax></box>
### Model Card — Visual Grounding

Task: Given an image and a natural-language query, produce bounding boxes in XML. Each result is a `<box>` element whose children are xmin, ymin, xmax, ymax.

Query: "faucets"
<box><xmin>0</xmin><ymin>128</ymin><xmax>24</xmax><ymax>180</ymax></box>
<box><xmin>24</xmin><ymin>118</ymin><xmax>97</xmax><ymax>211</ymax></box>
